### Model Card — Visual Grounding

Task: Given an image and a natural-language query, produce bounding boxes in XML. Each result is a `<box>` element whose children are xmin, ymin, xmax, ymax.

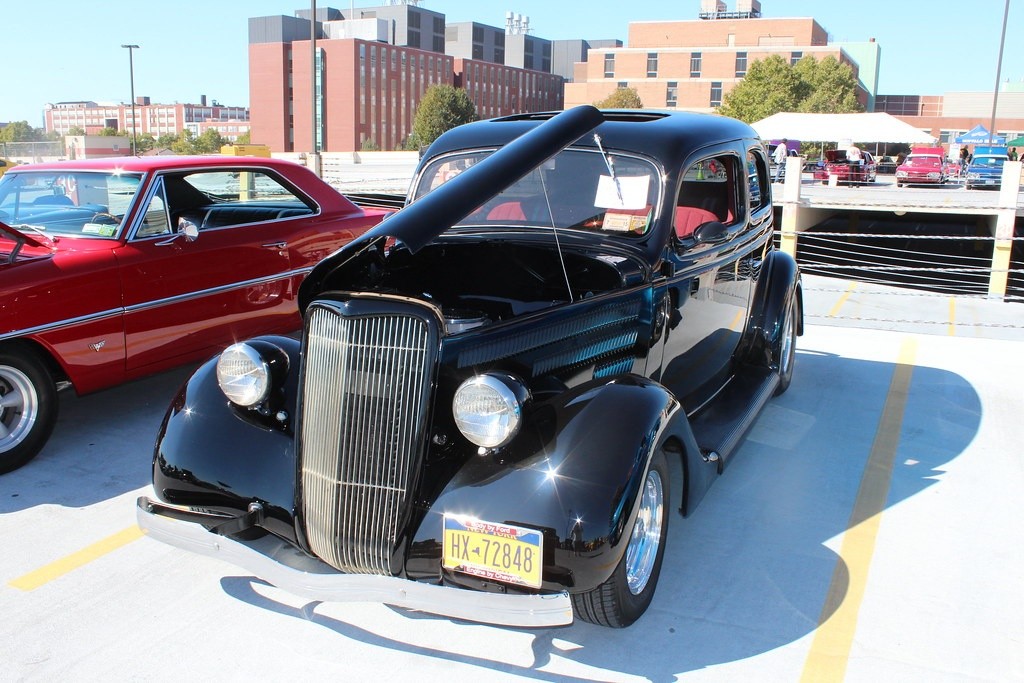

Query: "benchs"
<box><xmin>173</xmin><ymin>207</ymin><xmax>312</xmax><ymax>230</ymax></box>
<box><xmin>487</xmin><ymin>200</ymin><xmax>718</xmax><ymax>244</ymax></box>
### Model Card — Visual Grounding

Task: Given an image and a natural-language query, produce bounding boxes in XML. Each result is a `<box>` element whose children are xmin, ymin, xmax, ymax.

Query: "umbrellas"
<box><xmin>1006</xmin><ymin>135</ymin><xmax>1024</xmax><ymax>147</ymax></box>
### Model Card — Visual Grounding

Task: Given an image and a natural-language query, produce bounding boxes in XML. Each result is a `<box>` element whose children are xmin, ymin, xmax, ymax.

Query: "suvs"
<box><xmin>134</xmin><ymin>103</ymin><xmax>806</xmax><ymax>629</ymax></box>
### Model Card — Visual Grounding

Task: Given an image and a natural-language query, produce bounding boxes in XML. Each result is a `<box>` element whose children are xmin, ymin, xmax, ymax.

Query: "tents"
<box><xmin>954</xmin><ymin>124</ymin><xmax>1005</xmax><ymax>169</ymax></box>
<box><xmin>749</xmin><ymin>112</ymin><xmax>938</xmax><ymax>161</ymax></box>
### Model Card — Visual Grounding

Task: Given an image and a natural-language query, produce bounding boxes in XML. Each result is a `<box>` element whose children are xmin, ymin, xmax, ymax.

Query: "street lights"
<box><xmin>122</xmin><ymin>44</ymin><xmax>141</xmax><ymax>156</ymax></box>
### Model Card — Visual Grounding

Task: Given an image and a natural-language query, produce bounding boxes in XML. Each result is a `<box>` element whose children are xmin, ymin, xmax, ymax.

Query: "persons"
<box><xmin>960</xmin><ymin>144</ymin><xmax>973</xmax><ymax>171</ymax></box>
<box><xmin>846</xmin><ymin>141</ymin><xmax>861</xmax><ymax>189</ymax></box>
<box><xmin>770</xmin><ymin>138</ymin><xmax>787</xmax><ymax>183</ymax></box>
<box><xmin>1008</xmin><ymin>147</ymin><xmax>1024</xmax><ymax>163</ymax></box>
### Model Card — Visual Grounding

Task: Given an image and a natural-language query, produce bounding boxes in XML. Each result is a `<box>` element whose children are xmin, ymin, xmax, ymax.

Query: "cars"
<box><xmin>813</xmin><ymin>148</ymin><xmax>878</xmax><ymax>185</ymax></box>
<box><xmin>965</xmin><ymin>144</ymin><xmax>1012</xmax><ymax>190</ymax></box>
<box><xmin>0</xmin><ymin>152</ymin><xmax>406</xmax><ymax>474</ymax></box>
<box><xmin>766</xmin><ymin>144</ymin><xmax>800</xmax><ymax>180</ymax></box>
<box><xmin>895</xmin><ymin>146</ymin><xmax>950</xmax><ymax>187</ymax></box>
